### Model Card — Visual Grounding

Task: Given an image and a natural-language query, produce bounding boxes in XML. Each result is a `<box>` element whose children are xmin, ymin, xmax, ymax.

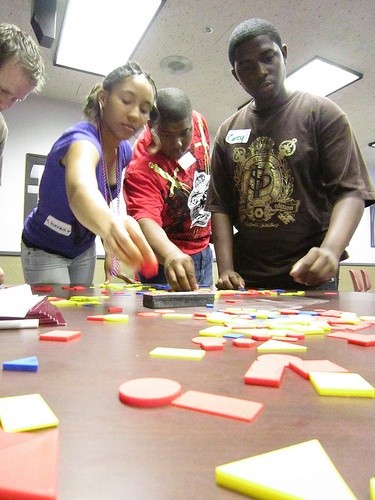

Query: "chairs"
<box><xmin>349</xmin><ymin>270</ymin><xmax>363</xmax><ymax>292</ymax></box>
<box><xmin>361</xmin><ymin>269</ymin><xmax>371</xmax><ymax>292</ymax></box>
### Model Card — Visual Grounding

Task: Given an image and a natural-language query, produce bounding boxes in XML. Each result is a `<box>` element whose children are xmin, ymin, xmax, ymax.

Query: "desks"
<box><xmin>0</xmin><ymin>284</ymin><xmax>375</xmax><ymax>500</ymax></box>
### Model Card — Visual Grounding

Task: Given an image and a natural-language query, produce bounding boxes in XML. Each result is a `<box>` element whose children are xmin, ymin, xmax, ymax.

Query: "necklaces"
<box><xmin>95</xmin><ymin>121</ymin><xmax>125</xmax><ymax>276</ymax></box>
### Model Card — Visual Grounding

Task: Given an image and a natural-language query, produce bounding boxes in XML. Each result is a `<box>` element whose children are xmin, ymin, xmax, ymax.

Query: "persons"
<box><xmin>19</xmin><ymin>59</ymin><xmax>158</xmax><ymax>288</ymax></box>
<box><xmin>120</xmin><ymin>86</ymin><xmax>218</xmax><ymax>290</ymax></box>
<box><xmin>0</xmin><ymin>22</ymin><xmax>48</xmax><ymax>287</ymax></box>
<box><xmin>202</xmin><ymin>17</ymin><xmax>375</xmax><ymax>293</ymax></box>
